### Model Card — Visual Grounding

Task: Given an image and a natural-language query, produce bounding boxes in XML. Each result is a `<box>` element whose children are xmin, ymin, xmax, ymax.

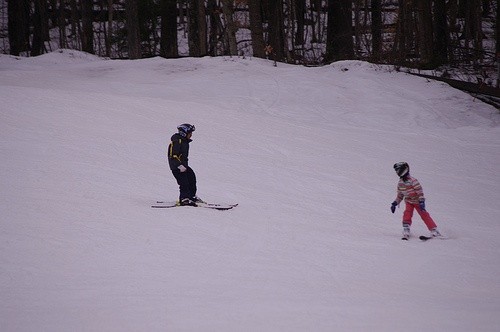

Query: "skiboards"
<box><xmin>152</xmin><ymin>201</ymin><xmax>238</xmax><ymax>210</ymax></box>
<box><xmin>401</xmin><ymin>235</ymin><xmax>433</xmax><ymax>240</ymax></box>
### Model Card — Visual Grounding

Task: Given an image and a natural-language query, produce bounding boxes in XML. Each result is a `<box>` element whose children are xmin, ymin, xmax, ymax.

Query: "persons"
<box><xmin>168</xmin><ymin>124</ymin><xmax>202</xmax><ymax>207</ymax></box>
<box><xmin>390</xmin><ymin>161</ymin><xmax>441</xmax><ymax>240</ymax></box>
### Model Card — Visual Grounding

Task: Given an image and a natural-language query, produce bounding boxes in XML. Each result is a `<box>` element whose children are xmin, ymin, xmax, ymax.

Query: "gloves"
<box><xmin>391</xmin><ymin>201</ymin><xmax>398</xmax><ymax>213</ymax></box>
<box><xmin>419</xmin><ymin>200</ymin><xmax>425</xmax><ymax>211</ymax></box>
<box><xmin>177</xmin><ymin>164</ymin><xmax>187</xmax><ymax>173</ymax></box>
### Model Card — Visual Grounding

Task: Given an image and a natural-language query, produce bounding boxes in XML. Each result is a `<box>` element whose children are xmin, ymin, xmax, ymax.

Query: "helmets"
<box><xmin>177</xmin><ymin>123</ymin><xmax>195</xmax><ymax>138</ymax></box>
<box><xmin>393</xmin><ymin>161</ymin><xmax>410</xmax><ymax>178</ymax></box>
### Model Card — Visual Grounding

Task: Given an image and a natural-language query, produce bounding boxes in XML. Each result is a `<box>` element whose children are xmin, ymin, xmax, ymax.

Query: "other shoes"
<box><xmin>403</xmin><ymin>228</ymin><xmax>411</xmax><ymax>237</ymax></box>
<box><xmin>431</xmin><ymin>227</ymin><xmax>440</xmax><ymax>237</ymax></box>
<box><xmin>179</xmin><ymin>196</ymin><xmax>203</xmax><ymax>206</ymax></box>
